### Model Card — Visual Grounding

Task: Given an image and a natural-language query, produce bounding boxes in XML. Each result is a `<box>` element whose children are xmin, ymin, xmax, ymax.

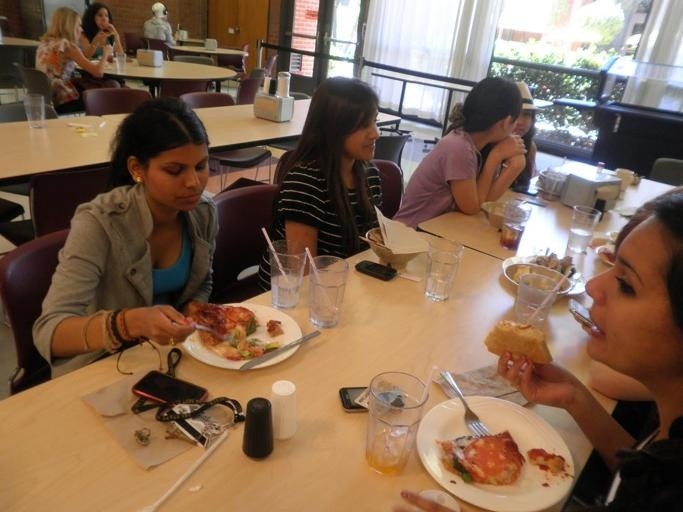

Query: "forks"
<box><xmin>168</xmin><ymin>320</ymin><xmax>239</xmax><ymax>347</ymax></box>
<box><xmin>437</xmin><ymin>370</ymin><xmax>493</xmax><ymax>439</ymax></box>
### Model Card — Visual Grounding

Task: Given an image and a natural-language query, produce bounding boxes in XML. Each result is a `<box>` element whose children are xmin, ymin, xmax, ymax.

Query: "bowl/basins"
<box><xmin>504</xmin><ymin>261</ymin><xmax>575</xmax><ymax>307</ymax></box>
<box><xmin>537</xmin><ymin>170</ymin><xmax>564</xmax><ymax>193</ymax></box>
<box><xmin>366</xmin><ymin>224</ymin><xmax>432</xmax><ymax>272</ymax></box>
<box><xmin>479</xmin><ymin>200</ymin><xmax>530</xmax><ymax>228</ymax></box>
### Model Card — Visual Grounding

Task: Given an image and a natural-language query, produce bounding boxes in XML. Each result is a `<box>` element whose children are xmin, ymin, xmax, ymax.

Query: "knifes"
<box><xmin>237</xmin><ymin>328</ymin><xmax>322</xmax><ymax>373</ymax></box>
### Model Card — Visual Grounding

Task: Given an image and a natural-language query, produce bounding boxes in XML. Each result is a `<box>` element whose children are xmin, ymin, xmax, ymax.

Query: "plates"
<box><xmin>595</xmin><ymin>244</ymin><xmax>617</xmax><ymax>267</ymax></box>
<box><xmin>415</xmin><ymin>393</ymin><xmax>576</xmax><ymax>512</ymax></box>
<box><xmin>606</xmin><ymin>230</ymin><xmax>622</xmax><ymax>242</ymax></box>
<box><xmin>91</xmin><ymin>60</ymin><xmax>100</xmax><ymax>65</ymax></box>
<box><xmin>181</xmin><ymin>301</ymin><xmax>304</xmax><ymax>372</ymax></box>
<box><xmin>502</xmin><ymin>254</ymin><xmax>589</xmax><ymax>296</ymax></box>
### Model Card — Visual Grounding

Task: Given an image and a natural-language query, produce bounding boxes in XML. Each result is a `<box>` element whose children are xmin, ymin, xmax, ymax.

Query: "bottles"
<box><xmin>174</xmin><ymin>23</ymin><xmax>181</xmax><ymax>40</ymax></box>
<box><xmin>263</xmin><ymin>76</ymin><xmax>271</xmax><ymax>95</ymax></box>
<box><xmin>269</xmin><ymin>78</ymin><xmax>277</xmax><ymax>96</ymax></box>
<box><xmin>595</xmin><ymin>161</ymin><xmax>606</xmax><ymax>174</ymax></box>
<box><xmin>589</xmin><ymin>198</ymin><xmax>607</xmax><ymax>224</ymax></box>
<box><xmin>241</xmin><ymin>397</ymin><xmax>275</xmax><ymax>461</ymax></box>
<box><xmin>276</xmin><ymin>71</ymin><xmax>290</xmax><ymax>99</ymax></box>
<box><xmin>268</xmin><ymin>379</ymin><xmax>297</xmax><ymax>442</ymax></box>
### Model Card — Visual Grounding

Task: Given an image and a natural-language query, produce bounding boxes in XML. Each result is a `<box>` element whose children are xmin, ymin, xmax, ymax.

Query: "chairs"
<box><xmin>648</xmin><ymin>157</ymin><xmax>683</xmax><ymax>184</ymax></box>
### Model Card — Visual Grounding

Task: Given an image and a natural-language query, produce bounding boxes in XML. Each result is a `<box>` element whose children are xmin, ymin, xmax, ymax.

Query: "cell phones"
<box><xmin>355</xmin><ymin>260</ymin><xmax>397</xmax><ymax>281</ymax></box>
<box><xmin>339</xmin><ymin>387</ymin><xmax>405</xmax><ymax>412</ymax></box>
<box><xmin>132</xmin><ymin>371</ymin><xmax>208</xmax><ymax>407</ymax></box>
<box><xmin>568</xmin><ymin>299</ymin><xmax>592</xmax><ymax>328</ymax></box>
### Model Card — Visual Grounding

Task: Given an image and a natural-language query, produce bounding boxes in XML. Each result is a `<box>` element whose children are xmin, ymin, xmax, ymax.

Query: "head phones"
<box><xmin>152</xmin><ymin>1</ymin><xmax>168</xmax><ymax>15</ymax></box>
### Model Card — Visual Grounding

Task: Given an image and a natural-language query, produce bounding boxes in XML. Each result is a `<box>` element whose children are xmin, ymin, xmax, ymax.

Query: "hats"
<box><xmin>515</xmin><ymin>82</ymin><xmax>553</xmax><ymax>111</ymax></box>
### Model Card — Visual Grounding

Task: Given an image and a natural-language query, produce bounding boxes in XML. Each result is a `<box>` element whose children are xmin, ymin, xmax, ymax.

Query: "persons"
<box><xmin>78</xmin><ymin>3</ymin><xmax>124</xmax><ymax>60</ymax></box>
<box><xmin>573</xmin><ymin>185</ymin><xmax>683</xmax><ymax>438</ymax></box>
<box><xmin>398</xmin><ymin>194</ymin><xmax>683</xmax><ymax>511</ymax></box>
<box><xmin>391</xmin><ymin>74</ymin><xmax>528</xmax><ymax>234</ymax></box>
<box><xmin>36</xmin><ymin>4</ymin><xmax>115</xmax><ymax>114</ymax></box>
<box><xmin>254</xmin><ymin>74</ymin><xmax>384</xmax><ymax>295</ymax></box>
<box><xmin>477</xmin><ymin>80</ymin><xmax>537</xmax><ymax>190</ymax></box>
<box><xmin>142</xmin><ymin>1</ymin><xmax>176</xmax><ymax>61</ymax></box>
<box><xmin>31</xmin><ymin>92</ymin><xmax>220</xmax><ymax>383</ymax></box>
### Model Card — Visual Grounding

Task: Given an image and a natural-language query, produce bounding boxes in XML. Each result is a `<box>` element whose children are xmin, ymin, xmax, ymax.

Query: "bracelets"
<box><xmin>80</xmin><ymin>309</ymin><xmax>150</xmax><ymax>352</ymax></box>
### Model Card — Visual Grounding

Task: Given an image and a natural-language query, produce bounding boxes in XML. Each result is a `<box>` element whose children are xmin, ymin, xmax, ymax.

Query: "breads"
<box><xmin>484</xmin><ymin>320</ymin><xmax>554</xmax><ymax>367</ymax></box>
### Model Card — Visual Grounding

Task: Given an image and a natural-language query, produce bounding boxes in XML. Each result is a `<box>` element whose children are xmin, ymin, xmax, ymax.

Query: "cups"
<box><xmin>105</xmin><ymin>47</ymin><xmax>114</xmax><ymax>64</ymax></box>
<box><xmin>614</xmin><ymin>167</ymin><xmax>634</xmax><ymax>201</ymax></box>
<box><xmin>501</xmin><ymin>199</ymin><xmax>533</xmax><ymax>251</ymax></box>
<box><xmin>424</xmin><ymin>236</ymin><xmax>465</xmax><ymax>307</ymax></box>
<box><xmin>412</xmin><ymin>489</ymin><xmax>461</xmax><ymax>512</ymax></box>
<box><xmin>22</xmin><ymin>93</ymin><xmax>46</xmax><ymax>131</ymax></box>
<box><xmin>513</xmin><ymin>270</ymin><xmax>559</xmax><ymax>333</ymax></box>
<box><xmin>363</xmin><ymin>368</ymin><xmax>429</xmax><ymax>478</ymax></box>
<box><xmin>565</xmin><ymin>204</ymin><xmax>603</xmax><ymax>254</ymax></box>
<box><xmin>306</xmin><ymin>255</ymin><xmax>349</xmax><ymax>328</ymax></box>
<box><xmin>541</xmin><ymin>165</ymin><xmax>567</xmax><ymax>202</ymax></box>
<box><xmin>266</xmin><ymin>238</ymin><xmax>310</xmax><ymax>310</ymax></box>
<box><xmin>115</xmin><ymin>52</ymin><xmax>127</xmax><ymax>73</ymax></box>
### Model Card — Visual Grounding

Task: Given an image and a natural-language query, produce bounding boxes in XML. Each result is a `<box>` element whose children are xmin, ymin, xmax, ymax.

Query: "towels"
<box><xmin>83</xmin><ymin>372</ymin><xmax>230</xmax><ymax>470</ymax></box>
